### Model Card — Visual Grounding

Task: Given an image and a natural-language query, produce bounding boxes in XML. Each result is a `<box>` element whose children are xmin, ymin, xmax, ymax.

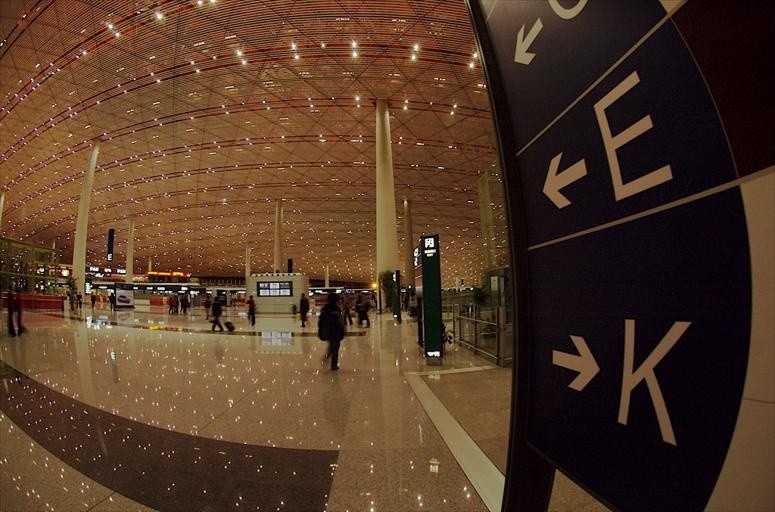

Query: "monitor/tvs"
<box><xmin>279</xmin><ymin>289</ymin><xmax>290</xmax><ymax>296</ymax></box>
<box><xmin>270</xmin><ymin>289</ymin><xmax>279</xmax><ymax>296</ymax></box>
<box><xmin>259</xmin><ymin>289</ymin><xmax>270</xmax><ymax>296</ymax></box>
<box><xmin>269</xmin><ymin>283</ymin><xmax>279</xmax><ymax>289</ymax></box>
<box><xmin>259</xmin><ymin>282</ymin><xmax>270</xmax><ymax>289</ymax></box>
<box><xmin>279</xmin><ymin>282</ymin><xmax>291</xmax><ymax>288</ymax></box>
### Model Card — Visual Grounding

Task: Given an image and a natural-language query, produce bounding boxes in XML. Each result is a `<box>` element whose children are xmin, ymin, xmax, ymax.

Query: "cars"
<box><xmin>118</xmin><ymin>295</ymin><xmax>130</xmax><ymax>303</ymax></box>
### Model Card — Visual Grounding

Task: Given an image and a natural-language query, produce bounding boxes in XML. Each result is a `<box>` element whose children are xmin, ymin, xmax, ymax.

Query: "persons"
<box><xmin>109</xmin><ymin>293</ymin><xmax>115</xmax><ymax>311</ymax></box>
<box><xmin>169</xmin><ymin>295</ymin><xmax>189</xmax><ymax>315</ymax></box>
<box><xmin>299</xmin><ymin>293</ymin><xmax>309</xmax><ymax>327</ymax></box>
<box><xmin>91</xmin><ymin>294</ymin><xmax>96</xmax><ymax>309</ymax></box>
<box><xmin>77</xmin><ymin>292</ymin><xmax>82</xmax><ymax>309</ymax></box>
<box><xmin>343</xmin><ymin>300</ymin><xmax>353</xmax><ymax>326</ymax></box>
<box><xmin>73</xmin><ymin>292</ymin><xmax>78</xmax><ymax>308</ymax></box>
<box><xmin>204</xmin><ymin>296</ymin><xmax>211</xmax><ymax>320</ymax></box>
<box><xmin>212</xmin><ymin>297</ymin><xmax>224</xmax><ymax>332</ymax></box>
<box><xmin>245</xmin><ymin>295</ymin><xmax>255</xmax><ymax>326</ymax></box>
<box><xmin>318</xmin><ymin>292</ymin><xmax>344</xmax><ymax>371</ymax></box>
<box><xmin>355</xmin><ymin>295</ymin><xmax>370</xmax><ymax>328</ymax></box>
